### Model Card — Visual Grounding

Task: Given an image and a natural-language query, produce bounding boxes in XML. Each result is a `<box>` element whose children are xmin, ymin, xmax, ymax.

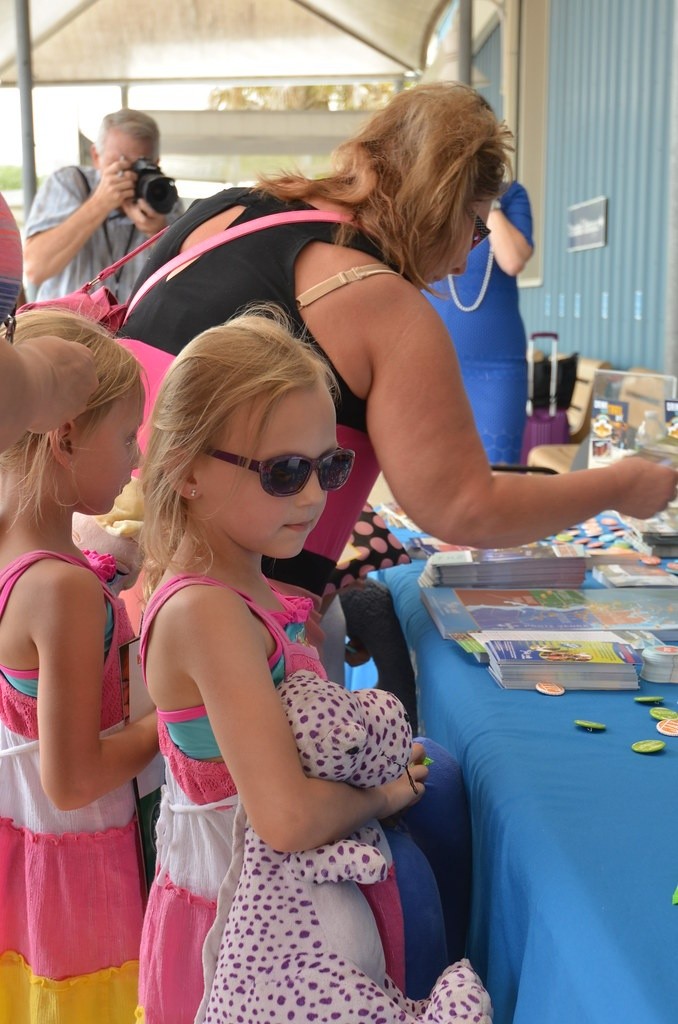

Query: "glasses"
<box><xmin>171</xmin><ymin>438</ymin><xmax>355</xmax><ymax>497</ymax></box>
<box><xmin>462</xmin><ymin>202</ymin><xmax>492</xmax><ymax>252</ymax></box>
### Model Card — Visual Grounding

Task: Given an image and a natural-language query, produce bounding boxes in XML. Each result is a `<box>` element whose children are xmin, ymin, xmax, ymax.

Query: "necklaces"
<box><xmin>448</xmin><ymin>245</ymin><xmax>493</xmax><ymax>313</ymax></box>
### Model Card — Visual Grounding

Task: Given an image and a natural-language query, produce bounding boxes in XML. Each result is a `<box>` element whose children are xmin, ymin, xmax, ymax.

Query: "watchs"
<box><xmin>491</xmin><ymin>200</ymin><xmax>501</xmax><ymax>209</ymax></box>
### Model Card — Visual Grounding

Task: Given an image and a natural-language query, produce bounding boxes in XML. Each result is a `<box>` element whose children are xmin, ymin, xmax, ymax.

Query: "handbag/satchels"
<box><xmin>13</xmin><ymin>224</ymin><xmax>172</xmax><ymax>335</ymax></box>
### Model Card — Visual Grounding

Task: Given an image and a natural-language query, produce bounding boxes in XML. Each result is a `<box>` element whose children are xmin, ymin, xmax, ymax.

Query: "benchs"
<box><xmin>526</xmin><ymin>368</ymin><xmax>668</xmax><ymax>477</ymax></box>
<box><xmin>547</xmin><ymin>353</ymin><xmax>612</xmax><ymax>444</ymax></box>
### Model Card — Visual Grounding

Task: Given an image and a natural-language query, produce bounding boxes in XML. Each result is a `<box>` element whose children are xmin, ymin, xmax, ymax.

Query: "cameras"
<box><xmin>127</xmin><ymin>158</ymin><xmax>178</xmax><ymax>215</ymax></box>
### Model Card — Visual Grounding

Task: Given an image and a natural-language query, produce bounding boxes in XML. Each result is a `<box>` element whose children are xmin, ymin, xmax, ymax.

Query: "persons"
<box><xmin>117</xmin><ymin>82</ymin><xmax>678</xmax><ymax>634</ymax></box>
<box><xmin>140</xmin><ymin>314</ymin><xmax>429</xmax><ymax>1024</ymax></box>
<box><xmin>420</xmin><ymin>179</ymin><xmax>534</xmax><ymax>467</ymax></box>
<box><xmin>0</xmin><ymin>310</ymin><xmax>159</xmax><ymax>1024</ymax></box>
<box><xmin>23</xmin><ymin>109</ymin><xmax>183</xmax><ymax>314</ymax></box>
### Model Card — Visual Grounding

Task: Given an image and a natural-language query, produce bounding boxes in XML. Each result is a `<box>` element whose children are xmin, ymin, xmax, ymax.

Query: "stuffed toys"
<box><xmin>191</xmin><ymin>669</ymin><xmax>494</xmax><ymax>1024</ymax></box>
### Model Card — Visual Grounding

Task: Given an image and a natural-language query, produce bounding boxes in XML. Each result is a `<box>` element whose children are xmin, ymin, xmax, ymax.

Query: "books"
<box><xmin>118</xmin><ymin>636</ymin><xmax>165</xmax><ymax>799</ymax></box>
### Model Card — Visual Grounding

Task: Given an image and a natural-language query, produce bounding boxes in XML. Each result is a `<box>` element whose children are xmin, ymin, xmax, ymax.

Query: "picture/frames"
<box><xmin>566</xmin><ymin>196</ymin><xmax>609</xmax><ymax>253</ymax></box>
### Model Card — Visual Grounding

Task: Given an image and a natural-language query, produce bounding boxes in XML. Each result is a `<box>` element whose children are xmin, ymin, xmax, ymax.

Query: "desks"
<box><xmin>342</xmin><ymin>506</ymin><xmax>678</xmax><ymax>1024</ymax></box>
